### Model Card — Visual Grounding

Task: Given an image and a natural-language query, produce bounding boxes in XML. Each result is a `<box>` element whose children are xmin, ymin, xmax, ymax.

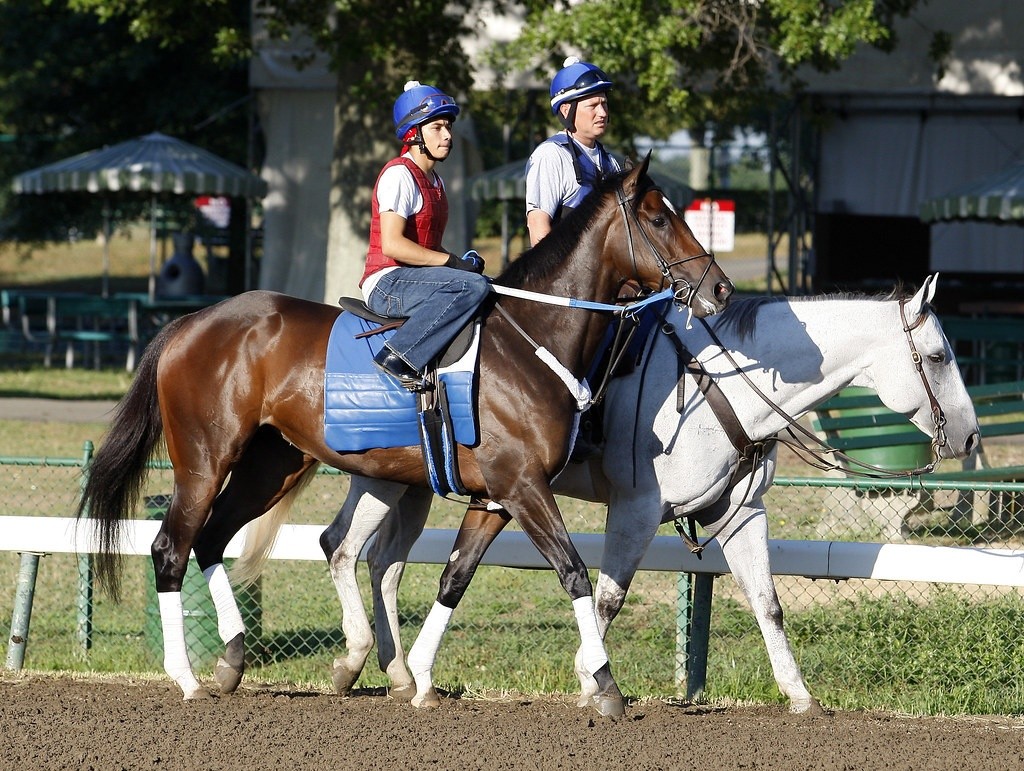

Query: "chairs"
<box><xmin>18</xmin><ymin>292</ymin><xmax>63</xmax><ymax>343</ymax></box>
<box><xmin>3</xmin><ymin>288</ymin><xmax>26</xmax><ymax>339</ymax></box>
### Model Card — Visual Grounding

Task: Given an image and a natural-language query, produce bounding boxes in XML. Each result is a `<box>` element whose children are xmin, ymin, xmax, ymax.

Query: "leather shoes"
<box><xmin>372</xmin><ymin>347</ymin><xmax>434</xmax><ymax>389</ymax></box>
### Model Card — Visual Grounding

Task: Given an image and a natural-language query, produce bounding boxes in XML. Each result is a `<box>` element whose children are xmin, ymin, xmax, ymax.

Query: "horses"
<box><xmin>317</xmin><ymin>271</ymin><xmax>982</xmax><ymax>715</ymax></box>
<box><xmin>66</xmin><ymin>149</ymin><xmax>731</xmax><ymax>718</ymax></box>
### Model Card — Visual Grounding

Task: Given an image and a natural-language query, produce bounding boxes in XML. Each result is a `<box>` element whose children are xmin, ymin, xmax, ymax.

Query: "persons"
<box><xmin>357</xmin><ymin>81</ymin><xmax>493</xmax><ymax>384</ymax></box>
<box><xmin>524</xmin><ymin>54</ymin><xmax>645</xmax><ymax>463</ymax></box>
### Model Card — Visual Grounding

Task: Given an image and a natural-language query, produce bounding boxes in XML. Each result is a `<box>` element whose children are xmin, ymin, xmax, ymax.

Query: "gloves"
<box><xmin>446</xmin><ymin>251</ymin><xmax>485</xmax><ymax>273</ymax></box>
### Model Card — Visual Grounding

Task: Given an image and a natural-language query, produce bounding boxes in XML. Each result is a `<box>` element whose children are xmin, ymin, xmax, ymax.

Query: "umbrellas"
<box><xmin>10</xmin><ymin>131</ymin><xmax>270</xmax><ymax>308</ymax></box>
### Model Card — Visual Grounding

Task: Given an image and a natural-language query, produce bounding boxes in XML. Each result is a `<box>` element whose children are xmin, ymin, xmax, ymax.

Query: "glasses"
<box><xmin>419</xmin><ymin>94</ymin><xmax>455</xmax><ymax>113</ymax></box>
<box><xmin>575</xmin><ymin>69</ymin><xmax>608</xmax><ymax>89</ymax></box>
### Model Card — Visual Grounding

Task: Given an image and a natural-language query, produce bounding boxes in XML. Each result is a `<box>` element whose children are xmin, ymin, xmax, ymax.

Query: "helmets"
<box><xmin>393</xmin><ymin>84</ymin><xmax>459</xmax><ymax>139</ymax></box>
<box><xmin>551</xmin><ymin>61</ymin><xmax>614</xmax><ymax>110</ymax></box>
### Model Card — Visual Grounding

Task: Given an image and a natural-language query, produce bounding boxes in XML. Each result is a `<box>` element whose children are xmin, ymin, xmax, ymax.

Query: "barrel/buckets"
<box><xmin>836</xmin><ymin>386</ymin><xmax>935</xmax><ymax>493</ymax></box>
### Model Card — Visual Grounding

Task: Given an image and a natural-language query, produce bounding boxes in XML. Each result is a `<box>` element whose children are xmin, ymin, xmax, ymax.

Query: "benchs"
<box><xmin>45</xmin><ymin>294</ymin><xmax>143</xmax><ymax>373</ymax></box>
<box><xmin>805</xmin><ymin>379</ymin><xmax>1024</xmax><ymax>543</ymax></box>
